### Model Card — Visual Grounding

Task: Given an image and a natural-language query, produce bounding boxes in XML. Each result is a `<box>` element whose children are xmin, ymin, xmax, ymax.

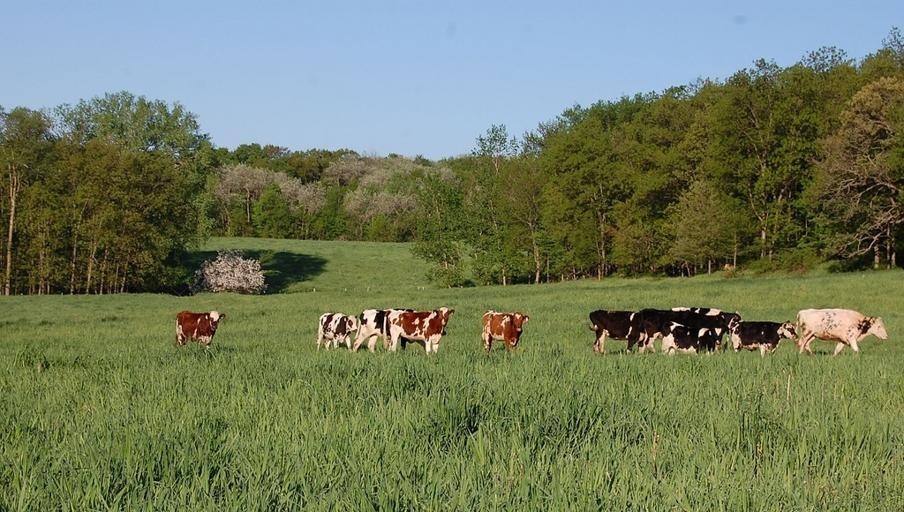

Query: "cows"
<box><xmin>589</xmin><ymin>307</ymin><xmax>742</xmax><ymax>356</ymax></box>
<box><xmin>731</xmin><ymin>320</ymin><xmax>799</xmax><ymax>357</ymax></box>
<box><xmin>480</xmin><ymin>309</ymin><xmax>530</xmax><ymax>353</ymax></box>
<box><xmin>317</xmin><ymin>306</ymin><xmax>455</xmax><ymax>356</ymax></box>
<box><xmin>175</xmin><ymin>310</ymin><xmax>226</xmax><ymax>348</ymax></box>
<box><xmin>795</xmin><ymin>308</ymin><xmax>888</xmax><ymax>358</ymax></box>
